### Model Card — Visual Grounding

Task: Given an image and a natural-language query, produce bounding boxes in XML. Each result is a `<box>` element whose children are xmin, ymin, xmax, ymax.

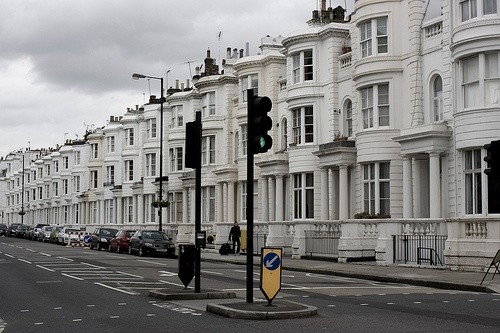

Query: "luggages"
<box><xmin>219</xmin><ymin>240</ymin><xmax>230</xmax><ymax>256</ymax></box>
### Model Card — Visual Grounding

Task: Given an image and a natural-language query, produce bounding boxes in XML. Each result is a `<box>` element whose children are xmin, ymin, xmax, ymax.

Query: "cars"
<box><xmin>0</xmin><ymin>224</ymin><xmax>7</xmax><ymax>237</ymax></box>
<box><xmin>50</xmin><ymin>226</ymin><xmax>64</xmax><ymax>243</ymax></box>
<box><xmin>38</xmin><ymin>225</ymin><xmax>53</xmax><ymax>242</ymax></box>
<box><xmin>16</xmin><ymin>224</ymin><xmax>30</xmax><ymax>239</ymax></box>
<box><xmin>128</xmin><ymin>230</ymin><xmax>175</xmax><ymax>257</ymax></box>
<box><xmin>109</xmin><ymin>231</ymin><xmax>137</xmax><ymax>252</ymax></box>
<box><xmin>7</xmin><ymin>223</ymin><xmax>19</xmax><ymax>238</ymax></box>
<box><xmin>58</xmin><ymin>228</ymin><xmax>81</xmax><ymax>245</ymax></box>
<box><xmin>89</xmin><ymin>228</ymin><xmax>118</xmax><ymax>251</ymax></box>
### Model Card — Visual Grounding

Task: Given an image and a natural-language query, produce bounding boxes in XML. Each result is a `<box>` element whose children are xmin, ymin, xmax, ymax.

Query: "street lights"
<box><xmin>131</xmin><ymin>73</ymin><xmax>168</xmax><ymax>232</ymax></box>
<box><xmin>8</xmin><ymin>153</ymin><xmax>26</xmax><ymax>223</ymax></box>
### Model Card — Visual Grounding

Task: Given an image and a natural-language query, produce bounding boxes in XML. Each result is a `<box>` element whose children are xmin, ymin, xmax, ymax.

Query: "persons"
<box><xmin>229</xmin><ymin>220</ymin><xmax>242</xmax><ymax>256</ymax></box>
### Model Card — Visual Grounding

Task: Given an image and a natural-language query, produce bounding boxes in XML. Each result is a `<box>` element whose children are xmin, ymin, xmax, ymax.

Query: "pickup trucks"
<box><xmin>25</xmin><ymin>224</ymin><xmax>50</xmax><ymax>240</ymax></box>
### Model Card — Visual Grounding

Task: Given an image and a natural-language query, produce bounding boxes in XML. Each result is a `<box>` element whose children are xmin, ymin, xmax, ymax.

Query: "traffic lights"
<box><xmin>252</xmin><ymin>96</ymin><xmax>274</xmax><ymax>154</ymax></box>
